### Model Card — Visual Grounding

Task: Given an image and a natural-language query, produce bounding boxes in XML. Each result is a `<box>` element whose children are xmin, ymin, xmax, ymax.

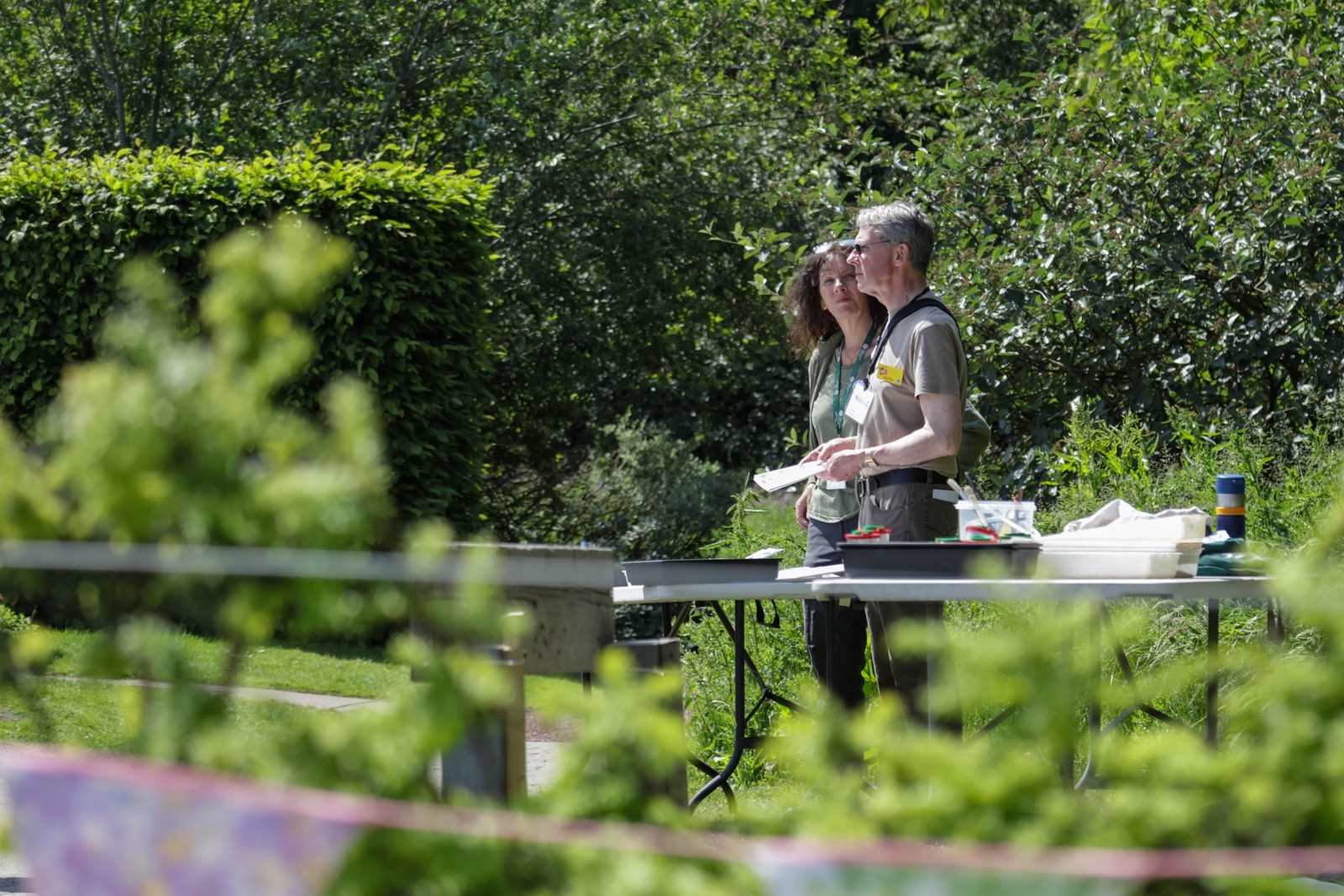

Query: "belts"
<box><xmin>858</xmin><ymin>467</ymin><xmax>949</xmax><ymax>498</ymax></box>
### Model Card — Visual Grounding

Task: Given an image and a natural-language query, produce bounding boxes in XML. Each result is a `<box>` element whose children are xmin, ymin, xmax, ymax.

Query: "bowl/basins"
<box><xmin>954</xmin><ymin>500</ymin><xmax>1037</xmax><ymax>542</ymax></box>
<box><xmin>1036</xmin><ymin>515</ymin><xmax>1208</xmax><ymax>578</ymax></box>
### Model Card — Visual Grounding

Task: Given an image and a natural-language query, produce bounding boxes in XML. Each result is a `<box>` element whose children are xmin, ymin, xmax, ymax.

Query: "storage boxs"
<box><xmin>625</xmin><ymin>556</ymin><xmax>784</xmax><ymax>589</ymax></box>
<box><xmin>835</xmin><ymin>500</ymin><xmax>1205</xmax><ymax>580</ymax></box>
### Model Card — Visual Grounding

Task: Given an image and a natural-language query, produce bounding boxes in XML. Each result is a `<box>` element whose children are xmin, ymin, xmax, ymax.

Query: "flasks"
<box><xmin>1216</xmin><ymin>474</ymin><xmax>1246</xmax><ymax>541</ymax></box>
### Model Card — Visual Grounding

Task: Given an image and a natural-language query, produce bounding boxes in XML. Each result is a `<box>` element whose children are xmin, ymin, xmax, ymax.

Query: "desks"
<box><xmin>612</xmin><ymin>584</ymin><xmax>819</xmax><ymax>813</ymax></box>
<box><xmin>812</xmin><ymin>577</ymin><xmax>1286</xmax><ymax>802</ymax></box>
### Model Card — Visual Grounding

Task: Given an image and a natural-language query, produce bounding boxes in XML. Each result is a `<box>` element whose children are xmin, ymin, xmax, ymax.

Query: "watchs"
<box><xmin>863</xmin><ymin>448</ymin><xmax>878</xmax><ymax>470</ymax></box>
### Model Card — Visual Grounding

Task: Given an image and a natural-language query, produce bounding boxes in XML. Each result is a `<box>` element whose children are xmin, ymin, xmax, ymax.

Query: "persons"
<box><xmin>797</xmin><ymin>202</ymin><xmax>968</xmax><ymax>712</ymax></box>
<box><xmin>784</xmin><ymin>238</ymin><xmax>992</xmax><ymax>738</ymax></box>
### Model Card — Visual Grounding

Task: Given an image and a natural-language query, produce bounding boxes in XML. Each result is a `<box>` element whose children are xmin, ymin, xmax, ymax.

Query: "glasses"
<box><xmin>812</xmin><ymin>239</ymin><xmax>855</xmax><ymax>254</ymax></box>
<box><xmin>852</xmin><ymin>239</ymin><xmax>910</xmax><ymax>256</ymax></box>
